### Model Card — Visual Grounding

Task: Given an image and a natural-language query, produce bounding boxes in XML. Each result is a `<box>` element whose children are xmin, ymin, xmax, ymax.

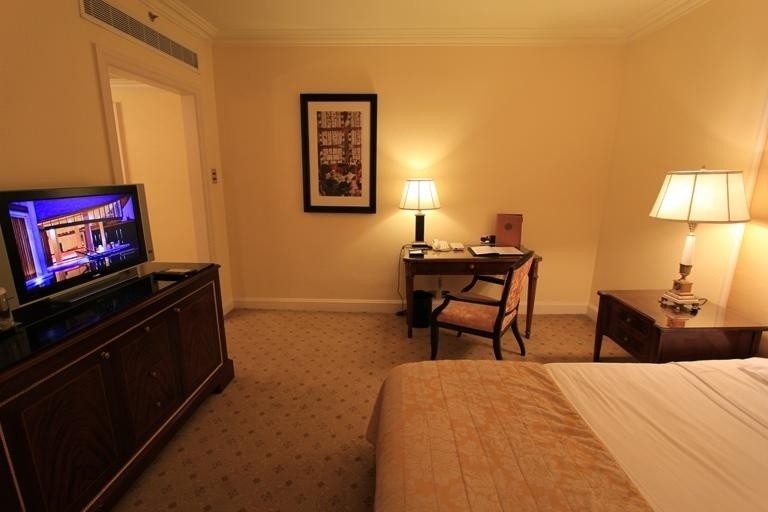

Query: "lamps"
<box><xmin>649</xmin><ymin>168</ymin><xmax>754</xmax><ymax>314</ymax></box>
<box><xmin>398</xmin><ymin>179</ymin><xmax>441</xmax><ymax>242</ymax></box>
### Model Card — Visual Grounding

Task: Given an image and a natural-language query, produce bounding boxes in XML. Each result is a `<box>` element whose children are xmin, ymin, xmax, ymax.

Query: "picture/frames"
<box><xmin>301</xmin><ymin>93</ymin><xmax>377</xmax><ymax>213</ymax></box>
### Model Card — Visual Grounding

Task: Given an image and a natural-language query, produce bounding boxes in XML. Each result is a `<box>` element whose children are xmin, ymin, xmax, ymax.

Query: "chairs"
<box><xmin>430</xmin><ymin>250</ymin><xmax>534</xmax><ymax>360</ymax></box>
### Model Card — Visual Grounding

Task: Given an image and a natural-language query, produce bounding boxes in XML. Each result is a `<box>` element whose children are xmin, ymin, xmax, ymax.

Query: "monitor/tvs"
<box><xmin>0</xmin><ymin>183</ymin><xmax>156</xmax><ymax>305</ymax></box>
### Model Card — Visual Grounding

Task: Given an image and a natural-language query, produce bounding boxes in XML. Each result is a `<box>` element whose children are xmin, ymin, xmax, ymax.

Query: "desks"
<box><xmin>403</xmin><ymin>242</ymin><xmax>542</xmax><ymax>338</ymax></box>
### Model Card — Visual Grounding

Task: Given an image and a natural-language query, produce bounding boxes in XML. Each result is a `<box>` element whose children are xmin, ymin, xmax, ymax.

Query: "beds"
<box><xmin>367</xmin><ymin>356</ymin><xmax>768</xmax><ymax>512</ymax></box>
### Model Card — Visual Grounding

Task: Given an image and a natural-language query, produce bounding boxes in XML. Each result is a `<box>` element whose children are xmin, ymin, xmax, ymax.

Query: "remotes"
<box><xmin>153</xmin><ymin>271</ymin><xmax>186</xmax><ymax>279</ymax></box>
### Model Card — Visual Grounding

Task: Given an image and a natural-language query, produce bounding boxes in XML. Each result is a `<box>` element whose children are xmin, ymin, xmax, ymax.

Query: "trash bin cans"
<box><xmin>407</xmin><ymin>290</ymin><xmax>433</xmax><ymax>328</ymax></box>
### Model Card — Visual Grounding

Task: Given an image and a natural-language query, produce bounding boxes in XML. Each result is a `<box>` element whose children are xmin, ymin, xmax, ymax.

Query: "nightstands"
<box><xmin>594</xmin><ymin>288</ymin><xmax>768</xmax><ymax>362</ymax></box>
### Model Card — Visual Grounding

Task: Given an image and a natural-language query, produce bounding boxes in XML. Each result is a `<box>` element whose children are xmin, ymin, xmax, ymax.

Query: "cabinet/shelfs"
<box><xmin>0</xmin><ymin>261</ymin><xmax>235</xmax><ymax>512</ymax></box>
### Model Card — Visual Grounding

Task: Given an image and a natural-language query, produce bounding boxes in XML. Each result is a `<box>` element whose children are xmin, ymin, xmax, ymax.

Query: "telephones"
<box><xmin>431</xmin><ymin>239</ymin><xmax>450</xmax><ymax>252</ymax></box>
<box><xmin>0</xmin><ymin>287</ymin><xmax>14</xmax><ymax>331</ymax></box>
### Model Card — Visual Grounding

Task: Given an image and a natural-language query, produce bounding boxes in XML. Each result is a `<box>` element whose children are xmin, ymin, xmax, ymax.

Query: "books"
<box><xmin>467</xmin><ymin>246</ymin><xmax>524</xmax><ymax>257</ymax></box>
